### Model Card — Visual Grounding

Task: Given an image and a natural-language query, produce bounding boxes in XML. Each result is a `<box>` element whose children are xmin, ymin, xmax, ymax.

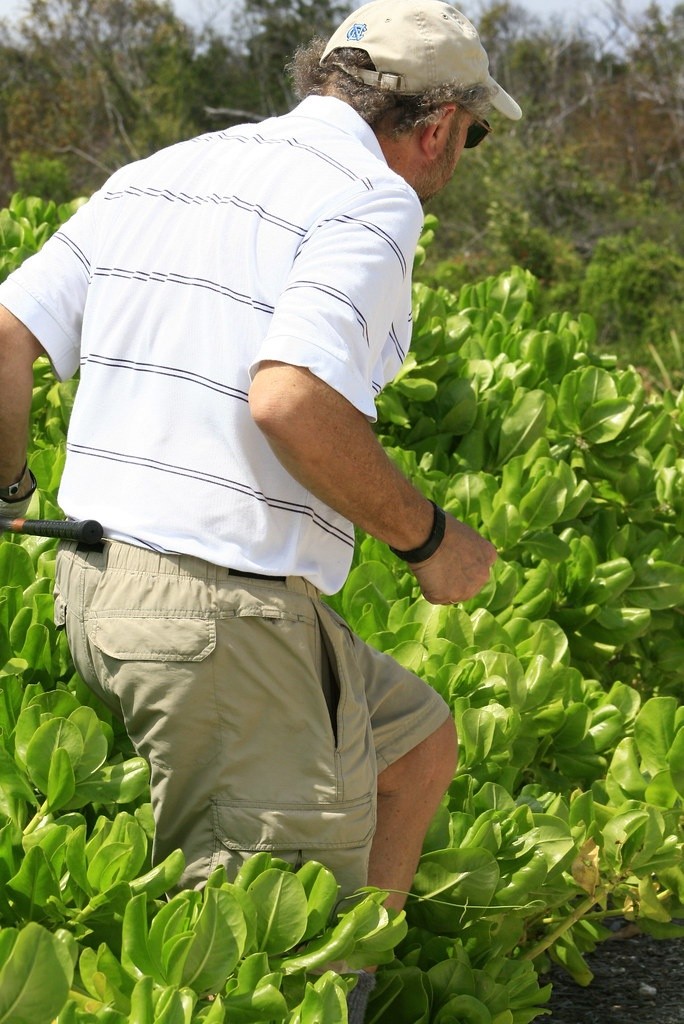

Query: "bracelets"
<box><xmin>0</xmin><ymin>458</ymin><xmax>28</xmax><ymax>497</ymax></box>
<box><xmin>388</xmin><ymin>498</ymin><xmax>447</xmax><ymax>564</ymax></box>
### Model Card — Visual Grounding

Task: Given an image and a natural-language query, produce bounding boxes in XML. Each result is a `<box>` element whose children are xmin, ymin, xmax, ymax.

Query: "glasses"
<box><xmin>446</xmin><ymin>99</ymin><xmax>491</xmax><ymax>148</ymax></box>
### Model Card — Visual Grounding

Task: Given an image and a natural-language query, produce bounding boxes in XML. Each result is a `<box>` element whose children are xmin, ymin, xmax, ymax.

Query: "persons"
<box><xmin>0</xmin><ymin>0</ymin><xmax>528</xmax><ymax>1024</ymax></box>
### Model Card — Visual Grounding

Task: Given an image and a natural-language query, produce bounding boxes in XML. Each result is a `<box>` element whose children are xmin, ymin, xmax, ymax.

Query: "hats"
<box><xmin>320</xmin><ymin>0</ymin><xmax>522</xmax><ymax>122</ymax></box>
